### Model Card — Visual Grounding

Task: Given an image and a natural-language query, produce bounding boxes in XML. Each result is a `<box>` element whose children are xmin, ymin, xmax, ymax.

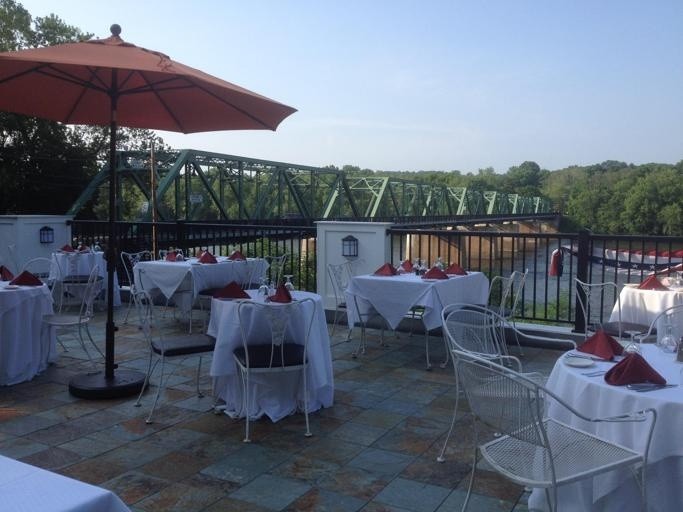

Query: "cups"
<box><xmin>673</xmin><ymin>271</ymin><xmax>683</xmax><ymax>292</ymax></box>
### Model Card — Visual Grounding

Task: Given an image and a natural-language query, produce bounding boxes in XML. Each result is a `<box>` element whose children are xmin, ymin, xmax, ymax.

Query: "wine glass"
<box><xmin>413</xmin><ymin>258</ymin><xmax>420</xmax><ymax>278</ymax></box>
<box><xmin>622</xmin><ymin>331</ymin><xmax>642</xmax><ymax>357</ymax></box>
<box><xmin>436</xmin><ymin>258</ymin><xmax>442</xmax><ymax>271</ymax></box>
<box><xmin>283</xmin><ymin>275</ymin><xmax>294</xmax><ymax>290</ymax></box>
<box><xmin>660</xmin><ymin>324</ymin><xmax>678</xmax><ymax>353</ymax></box>
<box><xmin>257</xmin><ymin>277</ymin><xmax>270</xmax><ymax>297</ymax></box>
<box><xmin>419</xmin><ymin>260</ymin><xmax>428</xmax><ymax>279</ymax></box>
<box><xmin>397</xmin><ymin>261</ymin><xmax>405</xmax><ymax>279</ymax></box>
<box><xmin>175</xmin><ymin>249</ymin><xmax>183</xmax><ymax>262</ymax></box>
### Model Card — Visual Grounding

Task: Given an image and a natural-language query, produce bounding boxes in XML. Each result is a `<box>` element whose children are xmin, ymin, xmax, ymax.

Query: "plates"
<box><xmin>4</xmin><ymin>285</ymin><xmax>19</xmax><ymax>289</ymax></box>
<box><xmin>565</xmin><ymin>357</ymin><xmax>594</xmax><ymax>367</ymax></box>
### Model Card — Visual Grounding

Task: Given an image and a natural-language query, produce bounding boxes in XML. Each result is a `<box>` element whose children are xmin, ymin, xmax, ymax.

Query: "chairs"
<box><xmin>197</xmin><ymin>257</ymin><xmax>260</xmax><ymax>337</ymax></box>
<box><xmin>52</xmin><ymin>252</ymin><xmax>104</xmax><ymax>315</ymax></box>
<box><xmin>572</xmin><ymin>277</ymin><xmax>659</xmax><ymax>341</ymax></box>
<box><xmin>326</xmin><ymin>261</ymin><xmax>400</xmax><ymax>347</ymax></box>
<box><xmin>21</xmin><ymin>257</ymin><xmax>68</xmax><ymax>353</ymax></box>
<box><xmin>129</xmin><ymin>283</ymin><xmax>217</xmax><ymax>424</ymax></box>
<box><xmin>232</xmin><ymin>296</ymin><xmax>315</xmax><ymax>443</ymax></box>
<box><xmin>239</xmin><ymin>252</ymin><xmax>289</xmax><ymax>291</ymax></box>
<box><xmin>440</xmin><ymin>275</ymin><xmax>513</xmax><ymax>369</ymax></box>
<box><xmin>436</xmin><ymin>302</ymin><xmax>579</xmax><ymax>465</ymax></box>
<box><xmin>158</xmin><ymin>249</ymin><xmax>205</xmax><ymax>322</ymax></box>
<box><xmin>449</xmin><ymin>349</ymin><xmax>658</xmax><ymax>512</ymax></box>
<box><xmin>458</xmin><ymin>267</ymin><xmax>529</xmax><ymax>364</ymax></box>
<box><xmin>624</xmin><ymin>305</ymin><xmax>683</xmax><ymax>344</ymax></box>
<box><xmin>37</xmin><ymin>263</ymin><xmax>106</xmax><ymax>371</ymax></box>
<box><xmin>119</xmin><ymin>250</ymin><xmax>155</xmax><ymax>328</ymax></box>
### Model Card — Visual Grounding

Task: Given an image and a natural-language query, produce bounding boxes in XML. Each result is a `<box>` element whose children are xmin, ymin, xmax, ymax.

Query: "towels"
<box><xmin>79</xmin><ymin>245</ymin><xmax>87</xmax><ymax>251</ymax></box>
<box><xmin>269</xmin><ymin>283</ymin><xmax>292</xmax><ymax>303</ymax></box>
<box><xmin>444</xmin><ymin>262</ymin><xmax>467</xmax><ymax>276</ymax></box>
<box><xmin>0</xmin><ymin>265</ymin><xmax>14</xmax><ymax>281</ymax></box>
<box><xmin>421</xmin><ymin>266</ymin><xmax>449</xmax><ymax>279</ymax></box>
<box><xmin>9</xmin><ymin>270</ymin><xmax>43</xmax><ymax>286</ymax></box>
<box><xmin>196</xmin><ymin>250</ymin><xmax>205</xmax><ymax>258</ymax></box>
<box><xmin>213</xmin><ymin>281</ymin><xmax>252</xmax><ymax>299</ymax></box>
<box><xmin>61</xmin><ymin>244</ymin><xmax>74</xmax><ymax>252</ymax></box>
<box><xmin>402</xmin><ymin>260</ymin><xmax>413</xmax><ymax>272</ymax></box>
<box><xmin>604</xmin><ymin>352</ymin><xmax>667</xmax><ymax>386</ymax></box>
<box><xmin>228</xmin><ymin>251</ymin><xmax>246</xmax><ymax>260</ymax></box>
<box><xmin>576</xmin><ymin>329</ymin><xmax>624</xmax><ymax>362</ymax></box>
<box><xmin>637</xmin><ymin>276</ymin><xmax>669</xmax><ymax>291</ymax></box>
<box><xmin>374</xmin><ymin>263</ymin><xmax>401</xmax><ymax>276</ymax></box>
<box><xmin>200</xmin><ymin>252</ymin><xmax>218</xmax><ymax>263</ymax></box>
<box><xmin>166</xmin><ymin>251</ymin><xmax>178</xmax><ymax>262</ymax></box>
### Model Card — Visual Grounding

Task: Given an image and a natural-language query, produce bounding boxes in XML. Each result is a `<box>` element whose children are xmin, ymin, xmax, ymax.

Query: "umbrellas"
<box><xmin>2</xmin><ymin>21</ymin><xmax>297</xmax><ymax>400</ymax></box>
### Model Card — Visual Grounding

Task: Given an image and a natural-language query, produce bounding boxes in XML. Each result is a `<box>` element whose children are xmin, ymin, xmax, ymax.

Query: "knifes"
<box><xmin>627</xmin><ymin>383</ymin><xmax>677</xmax><ymax>393</ymax></box>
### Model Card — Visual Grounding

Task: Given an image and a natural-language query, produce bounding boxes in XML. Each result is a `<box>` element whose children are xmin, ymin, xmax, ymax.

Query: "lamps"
<box><xmin>39</xmin><ymin>226</ymin><xmax>55</xmax><ymax>243</ymax></box>
<box><xmin>341</xmin><ymin>235</ymin><xmax>359</xmax><ymax>257</ymax></box>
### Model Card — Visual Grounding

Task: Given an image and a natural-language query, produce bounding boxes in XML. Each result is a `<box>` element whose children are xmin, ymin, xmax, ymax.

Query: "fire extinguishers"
<box><xmin>549</xmin><ymin>247</ymin><xmax>564</xmax><ymax>277</ymax></box>
